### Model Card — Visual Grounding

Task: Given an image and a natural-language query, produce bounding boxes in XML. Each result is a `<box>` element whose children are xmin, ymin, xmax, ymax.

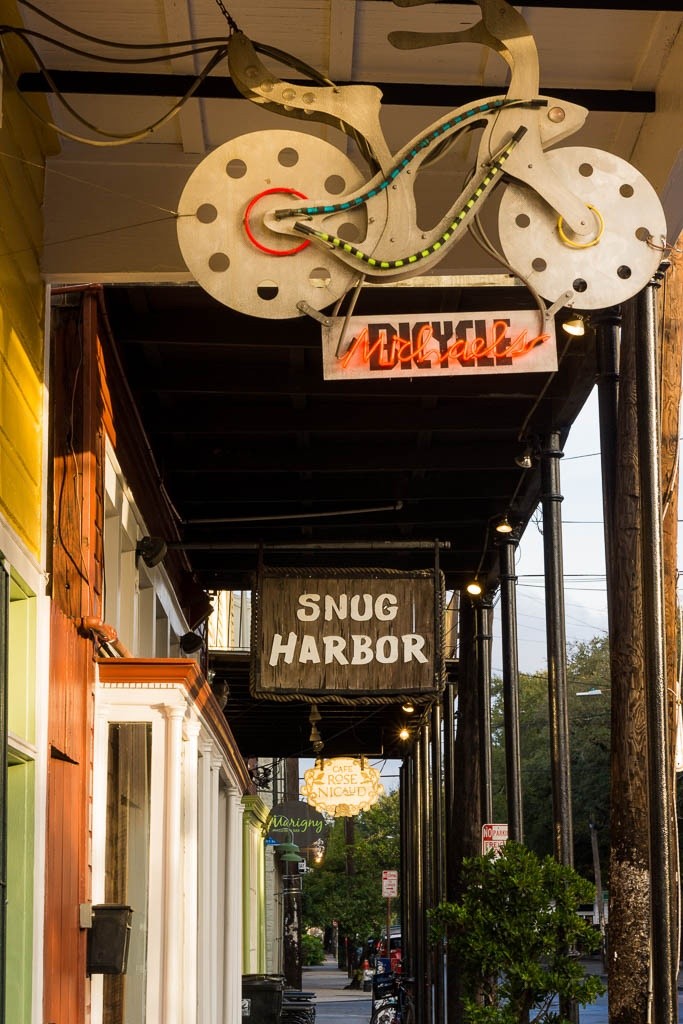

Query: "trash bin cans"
<box><xmin>242</xmin><ymin>974</ymin><xmax>282</xmax><ymax>1024</ymax></box>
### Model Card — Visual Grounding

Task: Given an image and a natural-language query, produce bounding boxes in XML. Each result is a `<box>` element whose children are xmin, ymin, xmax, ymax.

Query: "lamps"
<box><xmin>515</xmin><ymin>446</ymin><xmax>534</xmax><ymax>468</ymax></box>
<box><xmin>179</xmin><ymin>631</ymin><xmax>203</xmax><ymax>653</ymax></box>
<box><xmin>135</xmin><ymin>535</ymin><xmax>167</xmax><ymax>568</ymax></box>
<box><xmin>467</xmin><ymin>580</ymin><xmax>482</xmax><ymax>594</ymax></box>
<box><xmin>402</xmin><ymin>702</ymin><xmax>414</xmax><ymax>712</ymax></box>
<box><xmin>309</xmin><ymin>704</ymin><xmax>325</xmax><ymax>751</ymax></box>
<box><xmin>562</xmin><ymin>317</ymin><xmax>586</xmax><ymax>335</ymax></box>
<box><xmin>496</xmin><ymin>514</ymin><xmax>513</xmax><ymax>532</ymax></box>
<box><xmin>271</xmin><ymin>815</ymin><xmax>302</xmax><ymax>860</ymax></box>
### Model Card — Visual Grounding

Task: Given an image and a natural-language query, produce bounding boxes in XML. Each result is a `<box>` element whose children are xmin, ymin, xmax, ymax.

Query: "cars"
<box><xmin>358</xmin><ymin>933</ymin><xmax>403</xmax><ymax>991</ymax></box>
